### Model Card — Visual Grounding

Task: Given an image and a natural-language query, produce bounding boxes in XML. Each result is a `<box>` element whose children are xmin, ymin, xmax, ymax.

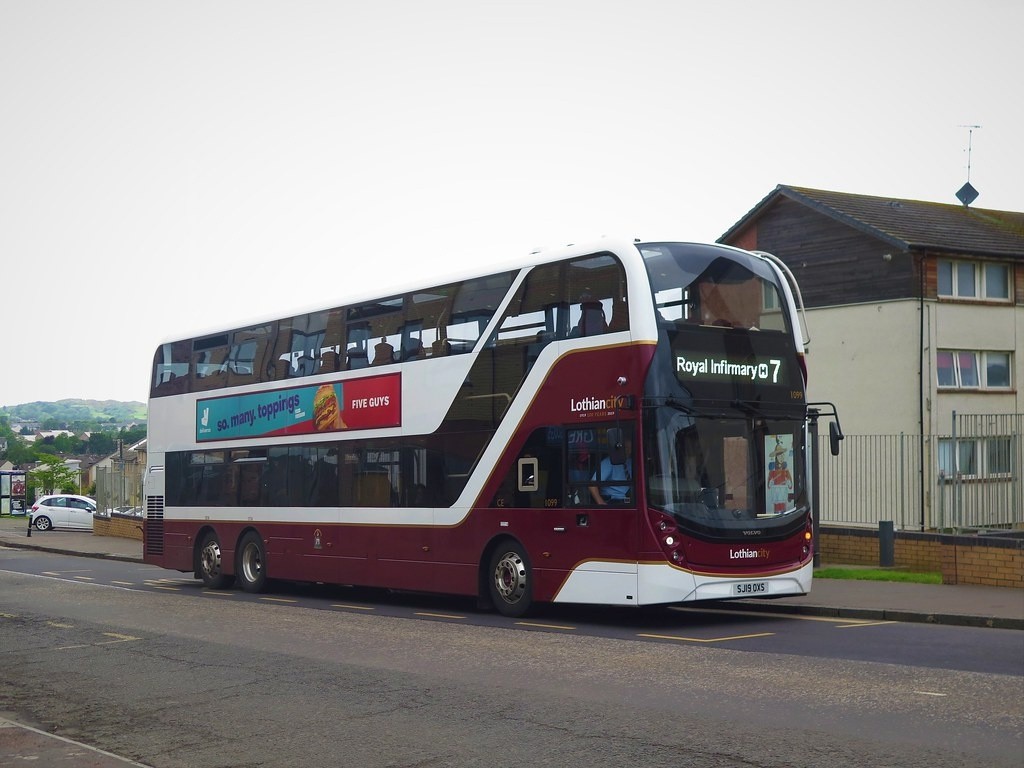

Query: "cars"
<box><xmin>31</xmin><ymin>494</ymin><xmax>123</xmax><ymax>531</ymax></box>
<box><xmin>105</xmin><ymin>505</ymin><xmax>144</xmax><ymax>517</ymax></box>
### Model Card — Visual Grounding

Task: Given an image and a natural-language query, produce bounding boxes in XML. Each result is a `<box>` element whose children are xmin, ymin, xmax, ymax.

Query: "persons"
<box><xmin>588</xmin><ymin>433</ymin><xmax>635</xmax><ymax>506</ymax></box>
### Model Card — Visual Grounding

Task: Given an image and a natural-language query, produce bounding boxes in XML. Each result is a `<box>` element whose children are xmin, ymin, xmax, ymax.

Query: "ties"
<box><xmin>624</xmin><ymin>463</ymin><xmax>631</xmax><ymax>481</ymax></box>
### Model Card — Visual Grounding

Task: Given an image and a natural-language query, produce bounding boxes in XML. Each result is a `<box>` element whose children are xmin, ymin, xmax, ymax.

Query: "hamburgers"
<box><xmin>313</xmin><ymin>384</ymin><xmax>339</xmax><ymax>432</ymax></box>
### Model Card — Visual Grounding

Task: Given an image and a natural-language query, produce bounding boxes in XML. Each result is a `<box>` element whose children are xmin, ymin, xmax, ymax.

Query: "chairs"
<box><xmin>157</xmin><ymin>338</ymin><xmax>451</xmax><ymax>396</ymax></box>
<box><xmin>580</xmin><ymin>297</ymin><xmax>629</xmax><ymax>336</ymax></box>
<box><xmin>674</xmin><ymin>318</ymin><xmax>732</xmax><ymax>328</ymax></box>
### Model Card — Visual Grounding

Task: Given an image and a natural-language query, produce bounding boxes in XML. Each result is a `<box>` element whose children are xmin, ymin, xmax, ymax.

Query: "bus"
<box><xmin>141</xmin><ymin>240</ymin><xmax>844</xmax><ymax>617</ymax></box>
